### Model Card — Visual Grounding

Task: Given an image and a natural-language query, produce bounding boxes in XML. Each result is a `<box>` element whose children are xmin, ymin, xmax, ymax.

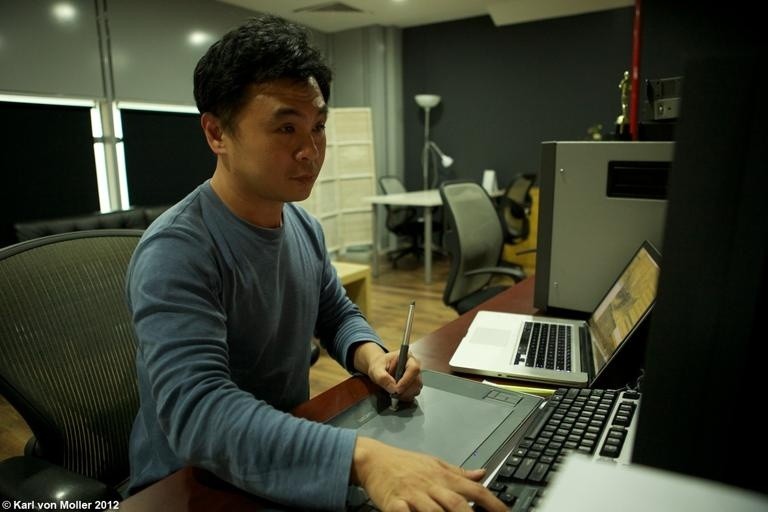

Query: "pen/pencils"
<box><xmin>390</xmin><ymin>301</ymin><xmax>416</xmax><ymax>412</ymax></box>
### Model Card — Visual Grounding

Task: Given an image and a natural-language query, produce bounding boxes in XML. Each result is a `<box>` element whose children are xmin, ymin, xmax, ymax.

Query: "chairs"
<box><xmin>439</xmin><ymin>174</ymin><xmax>536</xmax><ymax>314</ymax></box>
<box><xmin>1</xmin><ymin>227</ymin><xmax>142</xmax><ymax>512</ymax></box>
<box><xmin>378</xmin><ymin>176</ymin><xmax>439</xmax><ymax>269</ymax></box>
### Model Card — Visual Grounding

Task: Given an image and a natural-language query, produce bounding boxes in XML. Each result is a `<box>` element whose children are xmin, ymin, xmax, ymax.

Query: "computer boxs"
<box><xmin>533</xmin><ymin>137</ymin><xmax>675</xmax><ymax>319</ymax></box>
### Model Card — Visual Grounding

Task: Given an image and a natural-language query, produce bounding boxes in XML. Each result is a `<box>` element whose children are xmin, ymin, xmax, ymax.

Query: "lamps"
<box><xmin>415</xmin><ymin>94</ymin><xmax>454</xmax><ymax>189</ymax></box>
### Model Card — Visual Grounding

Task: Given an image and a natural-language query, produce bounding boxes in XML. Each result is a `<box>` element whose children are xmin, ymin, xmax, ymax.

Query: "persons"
<box><xmin>125</xmin><ymin>16</ymin><xmax>513</xmax><ymax>512</ymax></box>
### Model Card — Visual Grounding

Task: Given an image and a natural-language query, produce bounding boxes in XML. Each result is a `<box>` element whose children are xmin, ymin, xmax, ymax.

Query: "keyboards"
<box><xmin>468</xmin><ymin>388</ymin><xmax>644</xmax><ymax>512</ymax></box>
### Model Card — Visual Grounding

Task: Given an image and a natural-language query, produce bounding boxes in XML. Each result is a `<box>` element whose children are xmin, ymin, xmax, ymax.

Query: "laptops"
<box><xmin>447</xmin><ymin>239</ymin><xmax>663</xmax><ymax>386</ymax></box>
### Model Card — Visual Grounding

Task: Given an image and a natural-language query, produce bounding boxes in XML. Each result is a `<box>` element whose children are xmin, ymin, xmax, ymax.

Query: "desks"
<box><xmin>360</xmin><ymin>188</ymin><xmax>506</xmax><ymax>285</ymax></box>
<box><xmin>329</xmin><ymin>260</ymin><xmax>372</xmax><ymax>321</ymax></box>
<box><xmin>107</xmin><ymin>274</ymin><xmax>589</xmax><ymax>512</ymax></box>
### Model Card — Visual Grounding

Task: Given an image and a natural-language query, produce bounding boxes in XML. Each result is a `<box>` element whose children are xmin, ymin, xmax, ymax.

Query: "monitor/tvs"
<box><xmin>111</xmin><ymin>100</ymin><xmax>217</xmax><ymax>210</ymax></box>
<box><xmin>0</xmin><ymin>94</ymin><xmax>111</xmax><ymax>247</ymax></box>
<box><xmin>634</xmin><ymin>0</ymin><xmax>768</xmax><ymax>499</ymax></box>
